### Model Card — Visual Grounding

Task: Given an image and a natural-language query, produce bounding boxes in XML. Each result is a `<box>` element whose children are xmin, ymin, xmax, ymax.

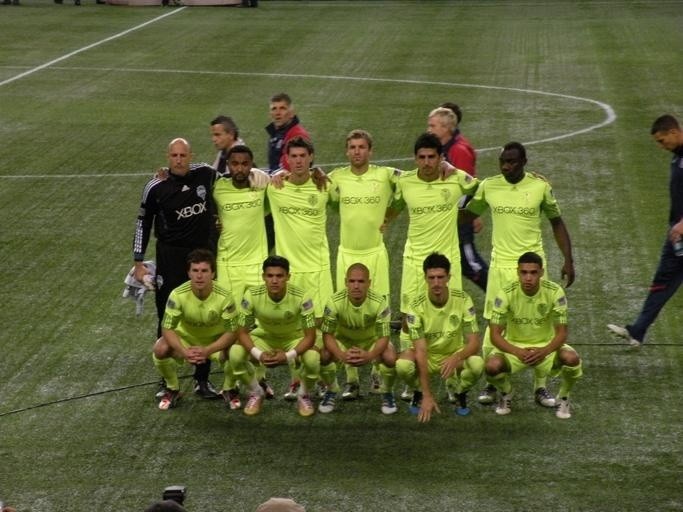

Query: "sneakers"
<box><xmin>478</xmin><ymin>383</ymin><xmax>496</xmax><ymax>404</ymax></box>
<box><xmin>408</xmin><ymin>388</ymin><xmax>423</xmax><ymax>414</ymax></box>
<box><xmin>553</xmin><ymin>392</ymin><xmax>571</xmax><ymax>418</ymax></box>
<box><xmin>342</xmin><ymin>374</ymin><xmax>399</xmax><ymax>415</ymax></box>
<box><xmin>399</xmin><ymin>382</ymin><xmax>416</xmax><ymax>400</ymax></box>
<box><xmin>452</xmin><ymin>388</ymin><xmax>472</xmax><ymax>416</ymax></box>
<box><xmin>217</xmin><ymin>378</ymin><xmax>274</xmax><ymax>416</ymax></box>
<box><xmin>494</xmin><ymin>386</ymin><xmax>516</xmax><ymax>415</ymax></box>
<box><xmin>534</xmin><ymin>386</ymin><xmax>556</xmax><ymax>408</ymax></box>
<box><xmin>285</xmin><ymin>373</ymin><xmax>340</xmax><ymax>416</ymax></box>
<box><xmin>443</xmin><ymin>380</ymin><xmax>456</xmax><ymax>402</ymax></box>
<box><xmin>605</xmin><ymin>323</ymin><xmax>643</xmax><ymax>349</ymax></box>
<box><xmin>154</xmin><ymin>372</ymin><xmax>219</xmax><ymax>410</ymax></box>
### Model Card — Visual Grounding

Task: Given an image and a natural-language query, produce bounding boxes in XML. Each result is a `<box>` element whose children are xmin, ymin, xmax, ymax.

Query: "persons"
<box><xmin>130</xmin><ymin>95</ymin><xmax>584</xmax><ymax>425</ymax></box>
<box><xmin>606</xmin><ymin>115</ymin><xmax>683</xmax><ymax>346</ymax></box>
<box><xmin>144</xmin><ymin>499</ymin><xmax>186</xmax><ymax>512</ymax></box>
<box><xmin>255</xmin><ymin>497</ymin><xmax>306</xmax><ymax>512</ymax></box>
